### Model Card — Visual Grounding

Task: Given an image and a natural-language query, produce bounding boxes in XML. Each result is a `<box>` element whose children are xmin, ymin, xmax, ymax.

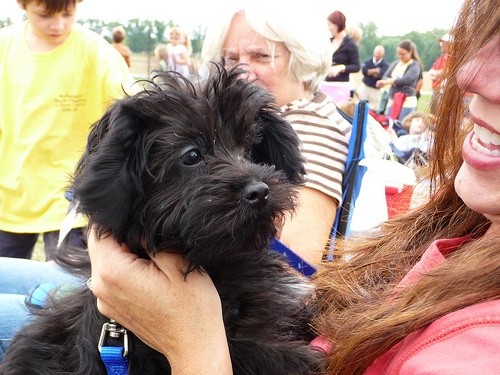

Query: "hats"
<box><xmin>436</xmin><ymin>34</ymin><xmax>455</xmax><ymax>42</ymax></box>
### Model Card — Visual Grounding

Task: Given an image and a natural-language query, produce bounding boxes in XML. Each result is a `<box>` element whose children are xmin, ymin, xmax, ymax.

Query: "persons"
<box><xmin>0</xmin><ymin>0</ymin><xmax>500</xmax><ymax>375</ymax></box>
<box><xmin>88</xmin><ymin>0</ymin><xmax>500</xmax><ymax>375</ymax></box>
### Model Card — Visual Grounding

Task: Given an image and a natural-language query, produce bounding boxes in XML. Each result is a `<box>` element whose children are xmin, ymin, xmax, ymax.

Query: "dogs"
<box><xmin>1</xmin><ymin>55</ymin><xmax>326</xmax><ymax>375</ymax></box>
